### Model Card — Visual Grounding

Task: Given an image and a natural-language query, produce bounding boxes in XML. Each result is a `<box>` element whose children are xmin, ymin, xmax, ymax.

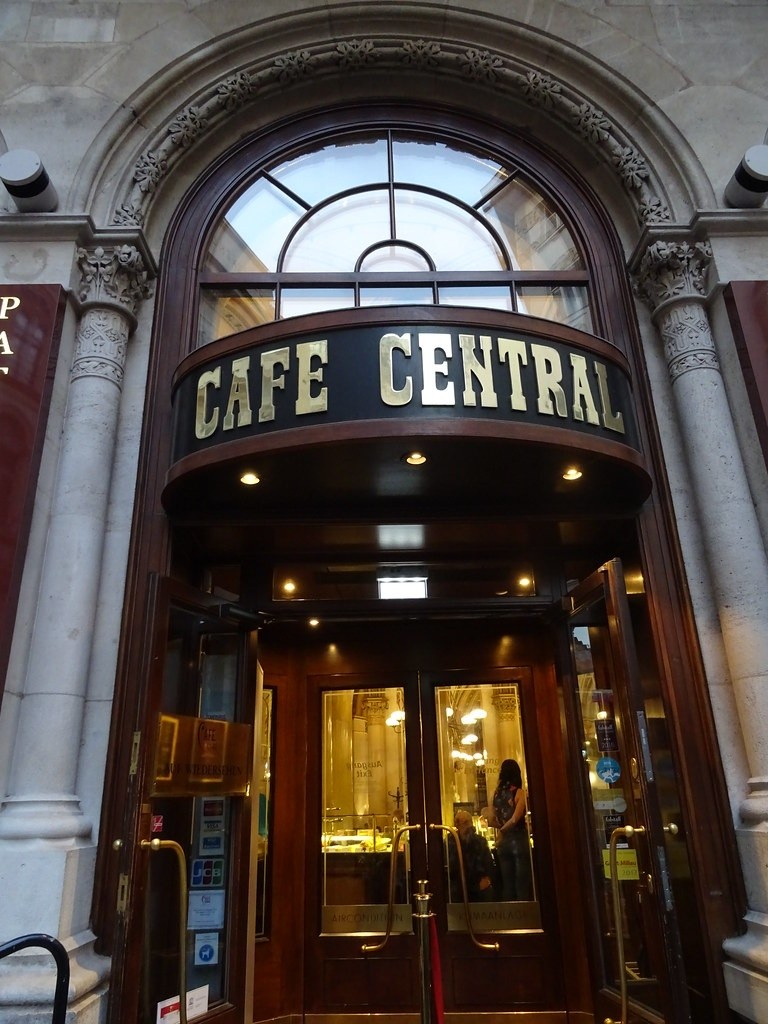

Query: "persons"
<box><xmin>446</xmin><ymin>811</ymin><xmax>497</xmax><ymax>902</ymax></box>
<box><xmin>487</xmin><ymin>758</ymin><xmax>534</xmax><ymax>901</ymax></box>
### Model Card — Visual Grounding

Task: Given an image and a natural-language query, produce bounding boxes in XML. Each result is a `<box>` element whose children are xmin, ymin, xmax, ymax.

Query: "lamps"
<box><xmin>401</xmin><ymin>443</ymin><xmax>435</xmax><ymax>467</ymax></box>
<box><xmin>382</xmin><ymin>684</ymin><xmax>490</xmax><ymax>767</ymax></box>
<box><xmin>563</xmin><ymin>460</ymin><xmax>583</xmax><ymax>482</ymax></box>
<box><xmin>239</xmin><ymin>470</ymin><xmax>261</xmax><ymax>486</ymax></box>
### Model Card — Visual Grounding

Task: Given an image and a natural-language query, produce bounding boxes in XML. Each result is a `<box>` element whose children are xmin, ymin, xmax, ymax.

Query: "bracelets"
<box><xmin>499</xmin><ymin>828</ymin><xmax>505</xmax><ymax>833</ymax></box>
